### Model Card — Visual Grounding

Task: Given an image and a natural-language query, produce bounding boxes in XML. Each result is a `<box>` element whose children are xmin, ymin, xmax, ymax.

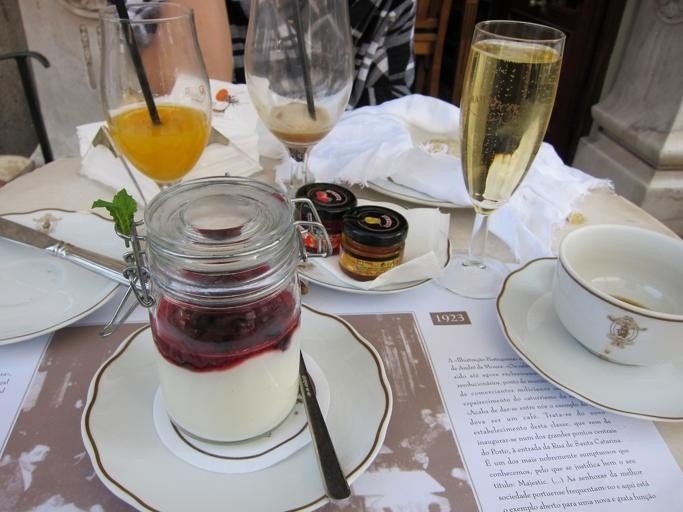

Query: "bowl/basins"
<box><xmin>553</xmin><ymin>221</ymin><xmax>683</xmax><ymax>370</ymax></box>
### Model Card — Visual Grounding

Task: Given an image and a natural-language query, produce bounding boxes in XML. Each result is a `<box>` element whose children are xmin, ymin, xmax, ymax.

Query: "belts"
<box><xmin>438</xmin><ymin>17</ymin><xmax>564</xmax><ymax>306</ymax></box>
<box><xmin>244</xmin><ymin>3</ymin><xmax>357</xmax><ymax>194</ymax></box>
<box><xmin>100</xmin><ymin>3</ymin><xmax>214</xmax><ymax>188</ymax></box>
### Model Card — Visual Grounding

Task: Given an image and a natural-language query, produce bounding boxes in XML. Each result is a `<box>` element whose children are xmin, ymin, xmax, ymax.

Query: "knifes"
<box><xmin>2</xmin><ymin>214</ymin><xmax>139</xmax><ymax>285</ymax></box>
<box><xmin>295</xmin><ymin>350</ymin><xmax>354</xmax><ymax>507</ymax></box>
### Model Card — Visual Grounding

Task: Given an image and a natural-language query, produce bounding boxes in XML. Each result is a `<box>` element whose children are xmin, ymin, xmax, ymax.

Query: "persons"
<box><xmin>112</xmin><ymin>0</ymin><xmax>417</xmax><ymax>105</ymax></box>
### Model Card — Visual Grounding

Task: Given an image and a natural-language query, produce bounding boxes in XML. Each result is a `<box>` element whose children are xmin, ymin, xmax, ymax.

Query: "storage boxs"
<box><xmin>412</xmin><ymin>0</ymin><xmax>457</xmax><ymax>98</ymax></box>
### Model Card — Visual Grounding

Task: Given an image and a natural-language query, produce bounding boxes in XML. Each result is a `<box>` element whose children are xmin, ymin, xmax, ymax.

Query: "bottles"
<box><xmin>337</xmin><ymin>205</ymin><xmax>411</xmax><ymax>283</ymax></box>
<box><xmin>292</xmin><ymin>181</ymin><xmax>358</xmax><ymax>257</ymax></box>
<box><xmin>116</xmin><ymin>175</ymin><xmax>334</xmax><ymax>445</ymax></box>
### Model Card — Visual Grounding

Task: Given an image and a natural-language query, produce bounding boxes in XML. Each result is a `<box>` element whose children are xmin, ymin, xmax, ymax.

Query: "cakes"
<box><xmin>338</xmin><ymin>205</ymin><xmax>408</xmax><ymax>281</ymax></box>
<box><xmin>293</xmin><ymin>183</ymin><xmax>357</xmax><ymax>255</ymax></box>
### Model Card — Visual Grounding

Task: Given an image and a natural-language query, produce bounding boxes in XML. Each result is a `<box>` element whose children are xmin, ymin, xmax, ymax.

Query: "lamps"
<box><xmin>0</xmin><ymin>51</ymin><xmax>55</xmax><ymax>187</ymax></box>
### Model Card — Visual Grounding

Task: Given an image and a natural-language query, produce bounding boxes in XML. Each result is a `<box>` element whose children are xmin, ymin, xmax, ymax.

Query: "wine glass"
<box><xmin>100</xmin><ymin>3</ymin><xmax>214</xmax><ymax>188</ymax></box>
<box><xmin>435</xmin><ymin>17</ymin><xmax>566</xmax><ymax>300</ymax></box>
<box><xmin>244</xmin><ymin>0</ymin><xmax>357</xmax><ymax>194</ymax></box>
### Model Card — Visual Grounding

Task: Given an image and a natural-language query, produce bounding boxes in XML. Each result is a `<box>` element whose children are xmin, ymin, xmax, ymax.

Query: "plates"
<box><xmin>364</xmin><ymin>148</ymin><xmax>481</xmax><ymax>211</ymax></box>
<box><xmin>297</xmin><ymin>196</ymin><xmax>454</xmax><ymax>298</ymax></box>
<box><xmin>2</xmin><ymin>203</ymin><xmax>134</xmax><ymax>349</ymax></box>
<box><xmin>494</xmin><ymin>255</ymin><xmax>682</xmax><ymax>423</ymax></box>
<box><xmin>77</xmin><ymin>301</ymin><xmax>394</xmax><ymax>509</ymax></box>
